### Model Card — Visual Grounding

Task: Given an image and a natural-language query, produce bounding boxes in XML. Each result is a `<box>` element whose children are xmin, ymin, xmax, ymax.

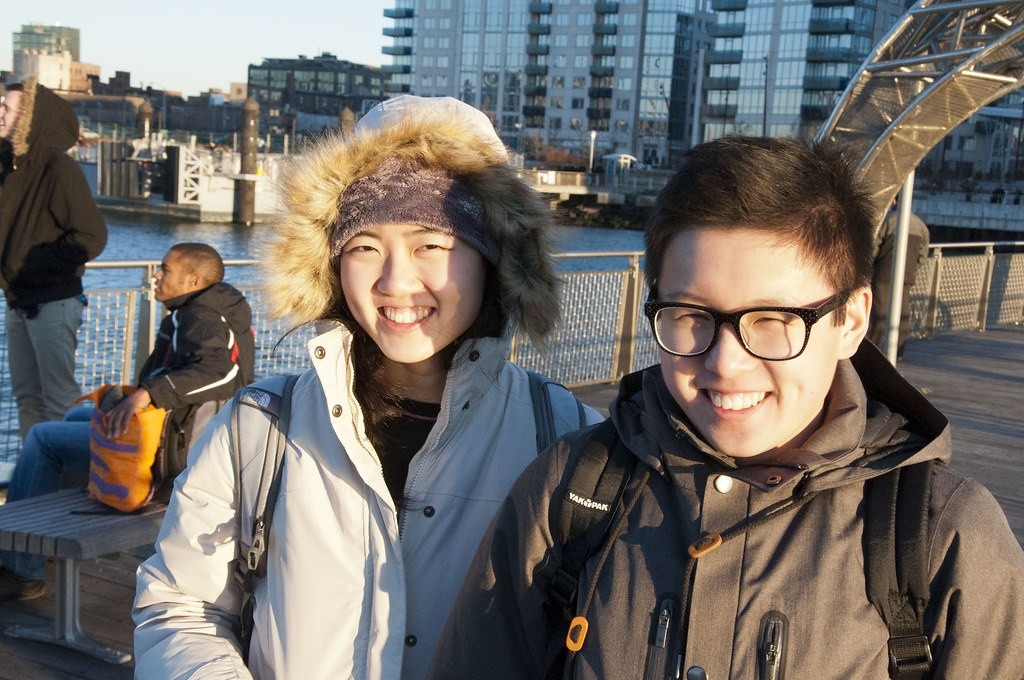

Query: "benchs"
<box><xmin>0</xmin><ymin>484</ymin><xmax>169</xmax><ymax>667</ymax></box>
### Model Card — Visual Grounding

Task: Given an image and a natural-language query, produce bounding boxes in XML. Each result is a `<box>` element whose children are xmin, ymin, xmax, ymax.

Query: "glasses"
<box><xmin>643</xmin><ymin>276</ymin><xmax>856</xmax><ymax>362</ymax></box>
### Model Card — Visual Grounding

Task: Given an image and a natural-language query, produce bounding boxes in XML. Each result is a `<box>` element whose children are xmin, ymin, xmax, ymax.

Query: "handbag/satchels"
<box><xmin>63</xmin><ymin>383</ymin><xmax>168</xmax><ymax>514</ymax></box>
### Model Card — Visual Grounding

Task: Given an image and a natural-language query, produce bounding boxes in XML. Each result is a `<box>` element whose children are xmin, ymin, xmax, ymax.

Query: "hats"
<box><xmin>329</xmin><ymin>154</ymin><xmax>503</xmax><ymax>274</ymax></box>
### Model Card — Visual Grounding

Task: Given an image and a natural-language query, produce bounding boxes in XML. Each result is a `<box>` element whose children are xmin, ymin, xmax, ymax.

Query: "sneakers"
<box><xmin>0</xmin><ymin>570</ymin><xmax>47</xmax><ymax>603</ymax></box>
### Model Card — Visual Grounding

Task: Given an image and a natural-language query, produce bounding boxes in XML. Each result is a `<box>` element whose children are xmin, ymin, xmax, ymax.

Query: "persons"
<box><xmin>0</xmin><ymin>76</ymin><xmax>108</xmax><ymax>443</ymax></box>
<box><xmin>423</xmin><ymin>134</ymin><xmax>1024</xmax><ymax>680</ymax></box>
<box><xmin>0</xmin><ymin>243</ymin><xmax>256</xmax><ymax>600</ymax></box>
<box><xmin>130</xmin><ymin>94</ymin><xmax>610</xmax><ymax>680</ymax></box>
